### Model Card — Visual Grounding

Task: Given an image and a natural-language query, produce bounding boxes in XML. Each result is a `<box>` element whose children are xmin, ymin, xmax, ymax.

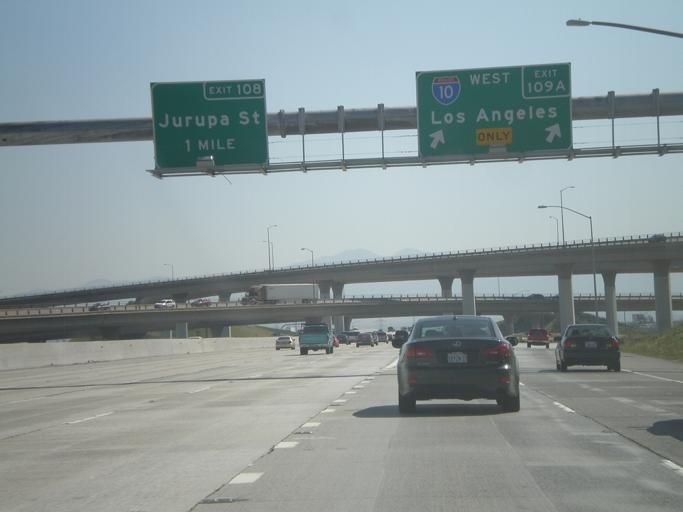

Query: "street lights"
<box><xmin>262</xmin><ymin>224</ymin><xmax>278</xmax><ymax>270</ymax></box>
<box><xmin>566</xmin><ymin>18</ymin><xmax>682</xmax><ymax>41</ymax></box>
<box><xmin>164</xmin><ymin>264</ymin><xmax>173</xmax><ymax>281</ymax></box>
<box><xmin>301</xmin><ymin>247</ymin><xmax>314</xmax><ymax>266</ymax></box>
<box><xmin>538</xmin><ymin>205</ymin><xmax>599</xmax><ymax>323</ymax></box>
<box><xmin>560</xmin><ymin>186</ymin><xmax>575</xmax><ymax>247</ymax></box>
<box><xmin>549</xmin><ymin>215</ymin><xmax>559</xmax><ymax>246</ymax></box>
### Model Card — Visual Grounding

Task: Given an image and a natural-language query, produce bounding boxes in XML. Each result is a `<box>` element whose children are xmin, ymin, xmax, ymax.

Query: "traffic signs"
<box><xmin>416</xmin><ymin>61</ymin><xmax>572</xmax><ymax>158</ymax></box>
<box><xmin>149</xmin><ymin>79</ymin><xmax>269</xmax><ymax>174</ymax></box>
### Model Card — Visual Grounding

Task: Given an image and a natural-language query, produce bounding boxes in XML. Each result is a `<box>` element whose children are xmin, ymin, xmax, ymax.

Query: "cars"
<box><xmin>192</xmin><ymin>299</ymin><xmax>211</xmax><ymax>306</ymax></box>
<box><xmin>529</xmin><ymin>294</ymin><xmax>544</xmax><ymax>299</ymax></box>
<box><xmin>90</xmin><ymin>303</ymin><xmax>111</xmax><ymax>311</ymax></box>
<box><xmin>334</xmin><ymin>329</ymin><xmax>394</xmax><ymax>348</ymax></box>
<box><xmin>554</xmin><ymin>324</ymin><xmax>622</xmax><ymax>372</ymax></box>
<box><xmin>393</xmin><ymin>314</ymin><xmax>521</xmax><ymax>413</ymax></box>
<box><xmin>276</xmin><ymin>336</ymin><xmax>295</xmax><ymax>350</ymax></box>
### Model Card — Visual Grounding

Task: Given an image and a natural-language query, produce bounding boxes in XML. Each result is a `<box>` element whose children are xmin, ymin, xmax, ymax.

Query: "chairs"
<box><xmin>571</xmin><ymin>329</ymin><xmax>581</xmax><ymax>337</ymax></box>
<box><xmin>425</xmin><ymin>330</ymin><xmax>437</xmax><ymax>337</ymax></box>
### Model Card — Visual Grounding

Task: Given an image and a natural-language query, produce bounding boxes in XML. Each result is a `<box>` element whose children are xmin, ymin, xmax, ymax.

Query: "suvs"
<box><xmin>154</xmin><ymin>299</ymin><xmax>176</xmax><ymax>309</ymax></box>
<box><xmin>527</xmin><ymin>328</ymin><xmax>550</xmax><ymax>349</ymax></box>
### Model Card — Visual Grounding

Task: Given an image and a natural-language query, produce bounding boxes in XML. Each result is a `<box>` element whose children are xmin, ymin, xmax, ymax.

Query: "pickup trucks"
<box><xmin>298</xmin><ymin>322</ymin><xmax>334</xmax><ymax>354</ymax></box>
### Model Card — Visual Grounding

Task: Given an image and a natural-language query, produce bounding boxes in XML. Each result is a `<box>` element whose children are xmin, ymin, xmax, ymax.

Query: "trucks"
<box><xmin>241</xmin><ymin>284</ymin><xmax>321</xmax><ymax>305</ymax></box>
